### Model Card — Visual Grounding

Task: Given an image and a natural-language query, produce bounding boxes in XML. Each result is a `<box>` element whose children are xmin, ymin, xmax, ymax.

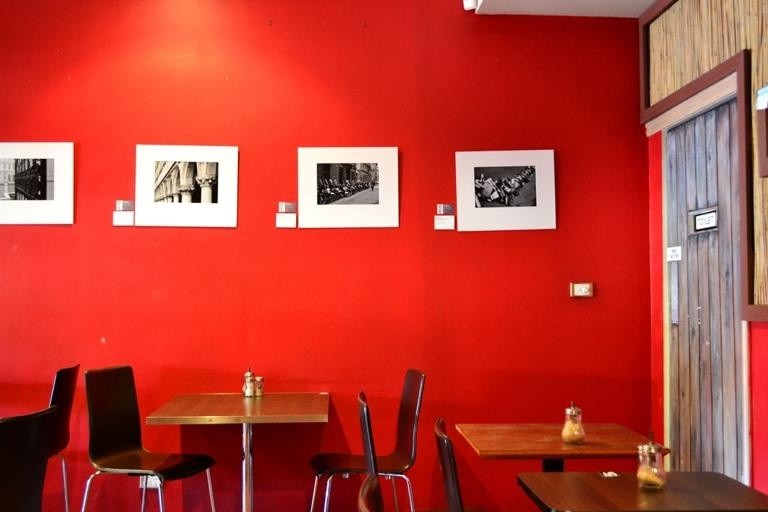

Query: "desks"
<box><xmin>146</xmin><ymin>391</ymin><xmax>331</xmax><ymax>512</ymax></box>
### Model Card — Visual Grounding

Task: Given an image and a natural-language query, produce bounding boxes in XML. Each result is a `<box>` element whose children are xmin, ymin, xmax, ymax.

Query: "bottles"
<box><xmin>242</xmin><ymin>368</ymin><xmax>264</xmax><ymax>396</ymax></box>
<box><xmin>561</xmin><ymin>402</ymin><xmax>585</xmax><ymax>443</ymax></box>
<box><xmin>635</xmin><ymin>432</ymin><xmax>667</xmax><ymax>490</ymax></box>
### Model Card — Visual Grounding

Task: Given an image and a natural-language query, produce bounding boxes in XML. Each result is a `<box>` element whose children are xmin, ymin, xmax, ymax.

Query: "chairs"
<box><xmin>355</xmin><ymin>390</ymin><xmax>382</xmax><ymax>512</ymax></box>
<box><xmin>0</xmin><ymin>364</ymin><xmax>81</xmax><ymax>511</ymax></box>
<box><xmin>309</xmin><ymin>368</ymin><xmax>426</xmax><ymax>512</ymax></box>
<box><xmin>1</xmin><ymin>366</ymin><xmax>463</xmax><ymax>509</ymax></box>
<box><xmin>81</xmin><ymin>365</ymin><xmax>217</xmax><ymax>512</ymax></box>
